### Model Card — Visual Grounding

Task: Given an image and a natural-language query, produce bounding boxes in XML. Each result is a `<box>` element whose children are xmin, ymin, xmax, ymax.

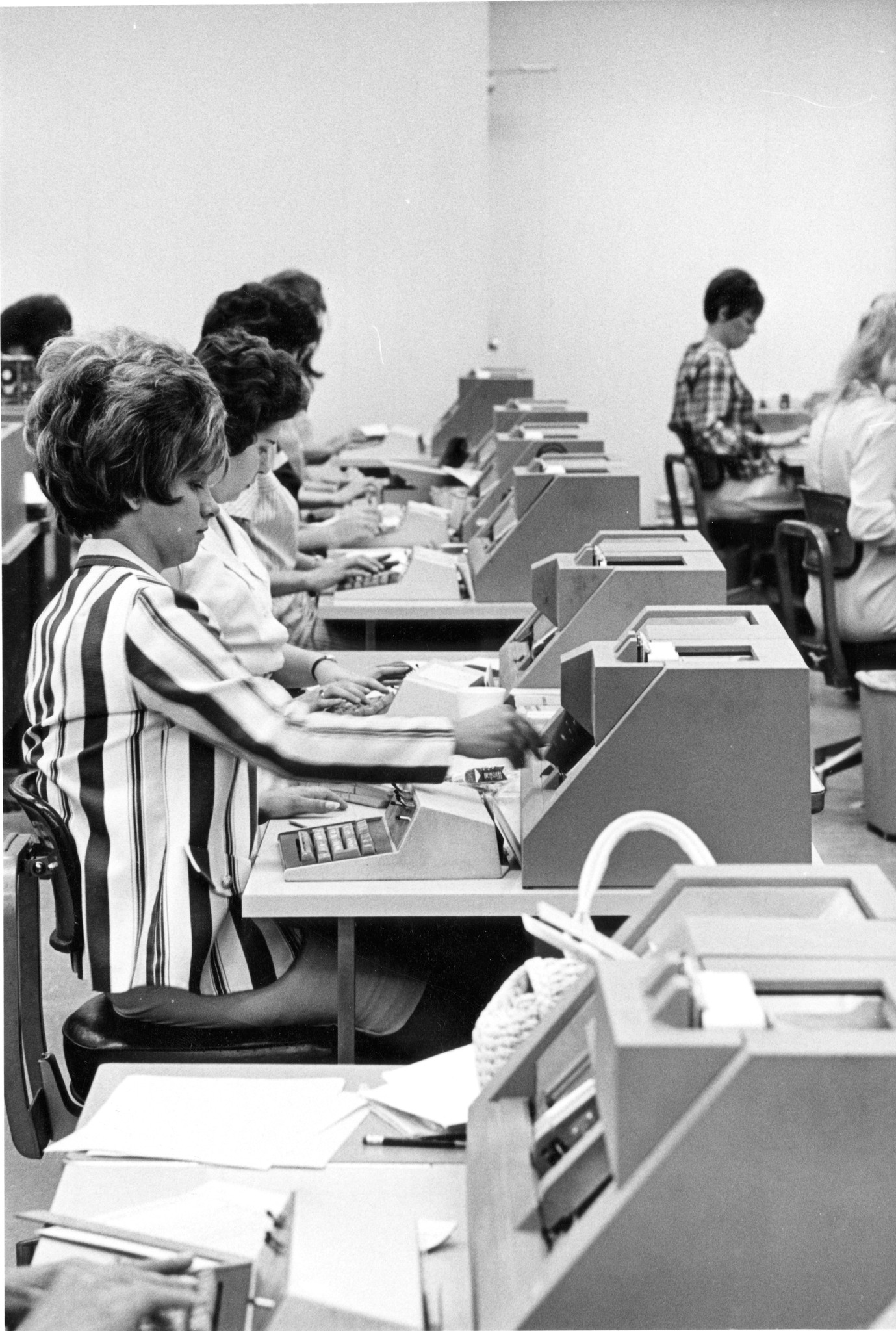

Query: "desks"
<box><xmin>331</xmin><ymin>429</ymin><xmax>467</xmax><ymax>557</ymax></box>
<box><xmin>318</xmin><ymin>548</ymin><xmax>538</xmax><ymax>651</ymax></box>
<box><xmin>27</xmin><ymin>1065</ymin><xmax>475</xmax><ymax>1331</ymax></box>
<box><xmin>755</xmin><ymin>409</ymin><xmax>812</xmax><ymax>469</ymax></box>
<box><xmin>242</xmin><ymin>649</ymin><xmax>653</xmax><ymax>1063</ymax></box>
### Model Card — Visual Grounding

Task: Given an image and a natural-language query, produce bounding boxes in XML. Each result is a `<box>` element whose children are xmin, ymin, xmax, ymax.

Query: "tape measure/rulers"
<box><xmin>14</xmin><ymin>1208</ymin><xmax>249</xmax><ymax>1263</ymax></box>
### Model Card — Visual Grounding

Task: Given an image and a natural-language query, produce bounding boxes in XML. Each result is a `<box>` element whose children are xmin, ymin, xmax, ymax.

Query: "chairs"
<box><xmin>664</xmin><ymin>420</ymin><xmax>807</xmax><ymax>598</ymax></box>
<box><xmin>4</xmin><ymin>772</ymin><xmax>339</xmax><ymax>1160</ymax></box>
<box><xmin>776</xmin><ymin>483</ymin><xmax>896</xmax><ymax>787</ymax></box>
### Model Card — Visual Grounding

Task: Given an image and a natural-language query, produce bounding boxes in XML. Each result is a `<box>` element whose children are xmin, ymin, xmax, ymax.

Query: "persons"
<box><xmin>20</xmin><ymin>337</ymin><xmax>548</xmax><ymax>1069</ymax></box>
<box><xmin>669</xmin><ymin>265</ymin><xmax>805</xmax><ymax>597</ymax></box>
<box><xmin>0</xmin><ymin>272</ymin><xmax>410</xmax><ymax>709</ymax></box>
<box><xmin>4</xmin><ymin>1244</ymin><xmax>211</xmax><ymax>1329</ymax></box>
<box><xmin>804</xmin><ymin>289</ymin><xmax>895</xmax><ymax>641</ymax></box>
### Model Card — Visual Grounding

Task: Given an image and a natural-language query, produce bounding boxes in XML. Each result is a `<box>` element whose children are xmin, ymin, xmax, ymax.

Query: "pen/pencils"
<box><xmin>420</xmin><ymin>1133</ymin><xmax>466</xmax><ymax>1141</ymax></box>
<box><xmin>363</xmin><ymin>1135</ymin><xmax>465</xmax><ymax>1149</ymax></box>
